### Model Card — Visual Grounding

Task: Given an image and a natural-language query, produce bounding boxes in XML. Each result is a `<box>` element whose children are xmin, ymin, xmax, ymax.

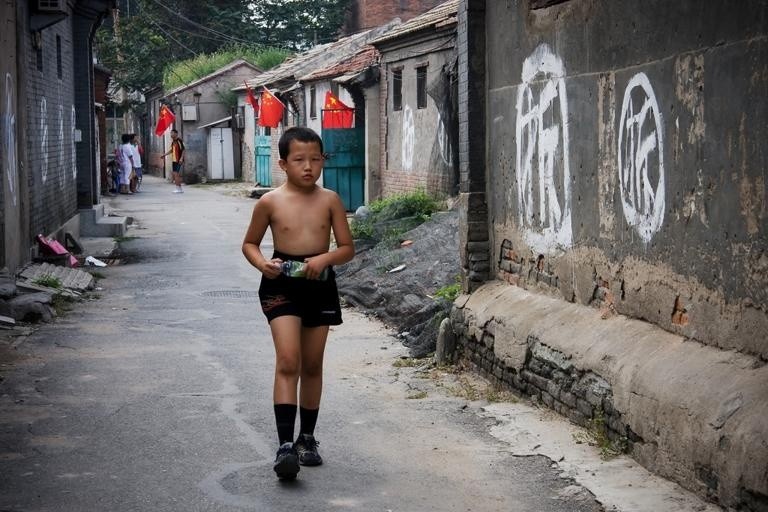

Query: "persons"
<box><xmin>117</xmin><ymin>134</ymin><xmax>134</xmax><ymax>194</ymax></box>
<box><xmin>130</xmin><ymin>134</ymin><xmax>144</xmax><ymax>192</ymax></box>
<box><xmin>242</xmin><ymin>126</ymin><xmax>355</xmax><ymax>481</ymax></box>
<box><xmin>161</xmin><ymin>129</ymin><xmax>186</xmax><ymax>193</ymax></box>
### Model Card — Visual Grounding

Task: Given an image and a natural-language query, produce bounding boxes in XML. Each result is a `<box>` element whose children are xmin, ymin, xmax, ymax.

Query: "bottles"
<box><xmin>273</xmin><ymin>260</ymin><xmax>328</xmax><ymax>281</ymax></box>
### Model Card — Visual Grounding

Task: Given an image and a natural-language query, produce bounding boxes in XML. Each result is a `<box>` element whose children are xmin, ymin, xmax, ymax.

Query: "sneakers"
<box><xmin>171</xmin><ymin>186</ymin><xmax>184</xmax><ymax>194</ymax></box>
<box><xmin>273</xmin><ymin>442</ymin><xmax>300</xmax><ymax>482</ymax></box>
<box><xmin>294</xmin><ymin>432</ymin><xmax>322</xmax><ymax>467</ymax></box>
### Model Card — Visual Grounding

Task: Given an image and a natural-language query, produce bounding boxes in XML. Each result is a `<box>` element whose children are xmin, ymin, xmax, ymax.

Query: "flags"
<box><xmin>245</xmin><ymin>87</ymin><xmax>259</xmax><ymax>112</ymax></box>
<box><xmin>258</xmin><ymin>87</ymin><xmax>285</xmax><ymax>127</ymax></box>
<box><xmin>154</xmin><ymin>104</ymin><xmax>176</xmax><ymax>136</ymax></box>
<box><xmin>323</xmin><ymin>91</ymin><xmax>353</xmax><ymax>129</ymax></box>
